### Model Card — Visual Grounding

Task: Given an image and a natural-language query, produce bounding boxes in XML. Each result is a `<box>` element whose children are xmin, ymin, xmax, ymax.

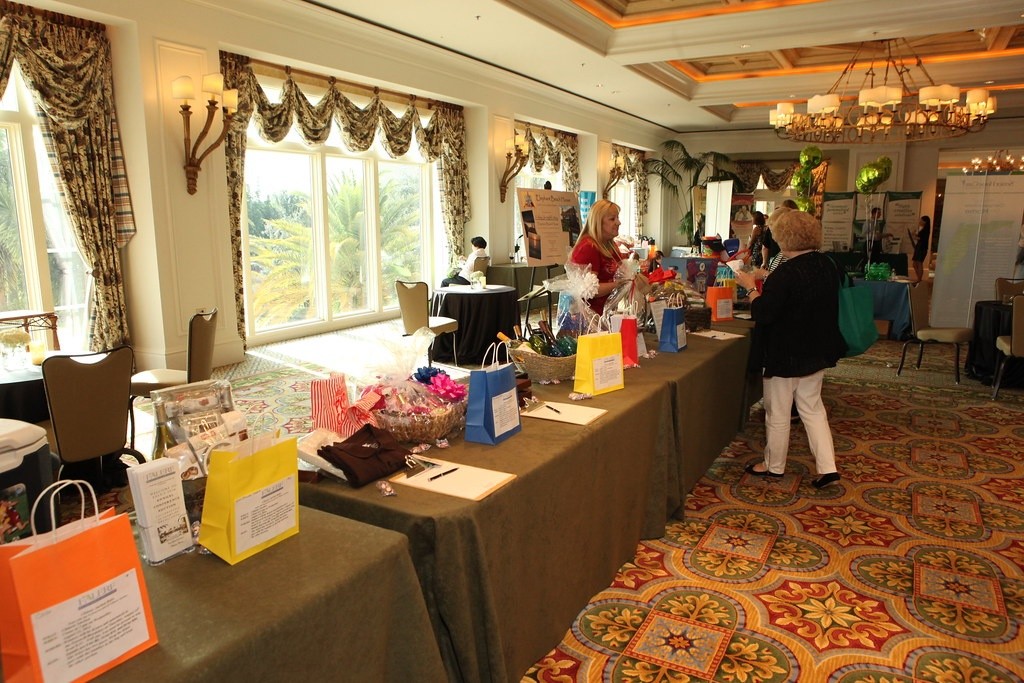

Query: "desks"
<box><xmin>89</xmin><ymin>503</ymin><xmax>457</xmax><ymax>683</ymax></box>
<box><xmin>711</xmin><ymin>309</ymin><xmax>763</xmax><ymax>405</ymax></box>
<box><xmin>828</xmin><ymin>250</ymin><xmax>917</xmax><ymax>338</ymax></box>
<box><xmin>300</xmin><ymin>379</ymin><xmax>674</xmax><ymax>683</ymax></box>
<box><xmin>0</xmin><ymin>310</ymin><xmax>60</xmax><ymax>351</ymax></box>
<box><xmin>964</xmin><ymin>300</ymin><xmax>1024</xmax><ymax>386</ymax></box>
<box><xmin>431</xmin><ymin>264</ymin><xmax>560</xmax><ymax>363</ymax></box>
<box><xmin>0</xmin><ymin>347</ymin><xmax>107</xmax><ymax>422</ymax></box>
<box><xmin>630</xmin><ymin>326</ymin><xmax>749</xmax><ymax>494</ymax></box>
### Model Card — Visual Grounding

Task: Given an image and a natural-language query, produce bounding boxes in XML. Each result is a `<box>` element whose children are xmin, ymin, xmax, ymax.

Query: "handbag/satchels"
<box><xmin>464</xmin><ymin>342</ymin><xmax>521</xmax><ymax>446</ymax></box>
<box><xmin>575</xmin><ymin>315</ymin><xmax>624</xmax><ymax>397</ymax></box>
<box><xmin>658</xmin><ymin>292</ymin><xmax>687</xmax><ymax>353</ymax></box>
<box><xmin>705</xmin><ymin>278</ymin><xmax>735</xmax><ymax>323</ymax></box>
<box><xmin>610</xmin><ymin>301</ymin><xmax>639</xmax><ymax>371</ymax></box>
<box><xmin>0</xmin><ymin>479</ymin><xmax>158</xmax><ymax>683</ymax></box>
<box><xmin>197</xmin><ymin>404</ymin><xmax>299</xmax><ymax>565</ymax></box>
<box><xmin>826</xmin><ymin>256</ymin><xmax>880</xmax><ymax>358</ymax></box>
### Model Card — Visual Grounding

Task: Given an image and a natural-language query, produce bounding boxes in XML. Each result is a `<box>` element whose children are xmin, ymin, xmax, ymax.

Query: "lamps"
<box><xmin>769</xmin><ymin>37</ymin><xmax>1024</xmax><ymax>173</ymax></box>
<box><xmin>172</xmin><ymin>73</ymin><xmax>239</xmax><ymax>195</ymax></box>
<box><xmin>496</xmin><ymin>135</ymin><xmax>529</xmax><ymax>203</ymax></box>
<box><xmin>603</xmin><ymin>156</ymin><xmax>625</xmax><ymax>199</ymax></box>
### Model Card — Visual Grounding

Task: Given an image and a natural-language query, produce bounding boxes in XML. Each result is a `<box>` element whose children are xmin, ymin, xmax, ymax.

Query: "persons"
<box><xmin>440</xmin><ymin>237</ymin><xmax>487</xmax><ymax>288</ymax></box>
<box><xmin>568</xmin><ymin>200</ymin><xmax>632</xmax><ymax>316</ymax></box>
<box><xmin>733</xmin><ymin>199</ymin><xmax>930</xmax><ymax>488</ymax></box>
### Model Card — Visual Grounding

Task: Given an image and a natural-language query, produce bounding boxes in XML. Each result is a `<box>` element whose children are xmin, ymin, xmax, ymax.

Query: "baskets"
<box><xmin>374</xmin><ymin>397</ymin><xmax>466</xmax><ymax>446</ymax></box>
<box><xmin>506</xmin><ymin>343</ymin><xmax>577</xmax><ymax>381</ymax></box>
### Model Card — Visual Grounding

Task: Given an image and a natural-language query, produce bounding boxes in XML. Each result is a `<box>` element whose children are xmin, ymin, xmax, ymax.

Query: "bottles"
<box><xmin>219</xmin><ymin>384</ymin><xmax>235</xmax><ymax>413</ymax></box>
<box><xmin>865</xmin><ymin>262</ymin><xmax>895</xmax><ymax>282</ymax></box>
<box><xmin>151</xmin><ymin>400</ymin><xmax>178</xmax><ymax>460</ymax></box>
<box><xmin>497</xmin><ymin>310</ymin><xmax>567</xmax><ymax>357</ymax></box>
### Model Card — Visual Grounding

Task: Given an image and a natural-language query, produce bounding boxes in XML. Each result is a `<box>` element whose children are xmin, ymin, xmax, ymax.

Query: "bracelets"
<box><xmin>746</xmin><ymin>287</ymin><xmax>757</xmax><ymax>296</ymax></box>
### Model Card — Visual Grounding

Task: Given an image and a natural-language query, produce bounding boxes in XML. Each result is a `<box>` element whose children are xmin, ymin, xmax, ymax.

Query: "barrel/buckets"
<box><xmin>0</xmin><ymin>418</ymin><xmax>56</xmax><ymax>546</ymax></box>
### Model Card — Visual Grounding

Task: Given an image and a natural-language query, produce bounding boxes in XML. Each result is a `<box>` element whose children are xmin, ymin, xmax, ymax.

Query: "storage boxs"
<box><xmin>0</xmin><ymin>418</ymin><xmax>54</xmax><ymax>540</ymax></box>
<box><xmin>661</xmin><ymin>256</ymin><xmax>718</xmax><ymax>292</ymax></box>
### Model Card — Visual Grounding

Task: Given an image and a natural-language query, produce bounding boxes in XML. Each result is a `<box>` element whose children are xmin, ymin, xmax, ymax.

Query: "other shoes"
<box><xmin>744</xmin><ymin>463</ymin><xmax>784</xmax><ymax>480</ymax></box>
<box><xmin>813</xmin><ymin>471</ymin><xmax>841</xmax><ymax>489</ymax></box>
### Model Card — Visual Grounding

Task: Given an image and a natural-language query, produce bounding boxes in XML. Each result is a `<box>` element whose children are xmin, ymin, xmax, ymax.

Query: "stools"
<box><xmin>896</xmin><ymin>327</ymin><xmax>973</xmax><ymax>386</ymax></box>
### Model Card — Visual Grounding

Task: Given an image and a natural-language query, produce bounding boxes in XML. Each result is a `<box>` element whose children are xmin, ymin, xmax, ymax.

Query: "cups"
<box><xmin>558</xmin><ymin>335</ymin><xmax>577</xmax><ymax>355</ymax></box>
<box><xmin>480</xmin><ymin>277</ymin><xmax>486</xmax><ymax>289</ymax></box>
<box><xmin>29</xmin><ymin>340</ymin><xmax>45</xmax><ymax>365</ymax></box>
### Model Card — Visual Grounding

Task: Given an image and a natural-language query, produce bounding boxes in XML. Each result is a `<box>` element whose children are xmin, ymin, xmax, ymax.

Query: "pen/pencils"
<box><xmin>427</xmin><ymin>467</ymin><xmax>459</xmax><ymax>481</ymax></box>
<box><xmin>712</xmin><ymin>334</ymin><xmax>725</xmax><ymax>337</ymax></box>
<box><xmin>546</xmin><ymin>405</ymin><xmax>560</xmax><ymax>413</ymax></box>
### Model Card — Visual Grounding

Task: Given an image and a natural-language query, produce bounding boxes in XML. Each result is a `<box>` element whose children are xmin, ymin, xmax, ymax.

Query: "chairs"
<box><xmin>42</xmin><ymin>307</ymin><xmax>218</xmax><ymax>520</ymax></box>
<box><xmin>396</xmin><ymin>281</ymin><xmax>458</xmax><ymax>369</ymax></box>
<box><xmin>992</xmin><ymin>277</ymin><xmax>1024</xmax><ymax>401</ymax></box>
<box><xmin>449</xmin><ymin>257</ymin><xmax>490</xmax><ymax>287</ymax></box>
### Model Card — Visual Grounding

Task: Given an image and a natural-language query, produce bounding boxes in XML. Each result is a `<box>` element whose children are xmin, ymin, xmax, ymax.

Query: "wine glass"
<box><xmin>509</xmin><ymin>252</ymin><xmax>514</xmax><ymax>264</ymax></box>
